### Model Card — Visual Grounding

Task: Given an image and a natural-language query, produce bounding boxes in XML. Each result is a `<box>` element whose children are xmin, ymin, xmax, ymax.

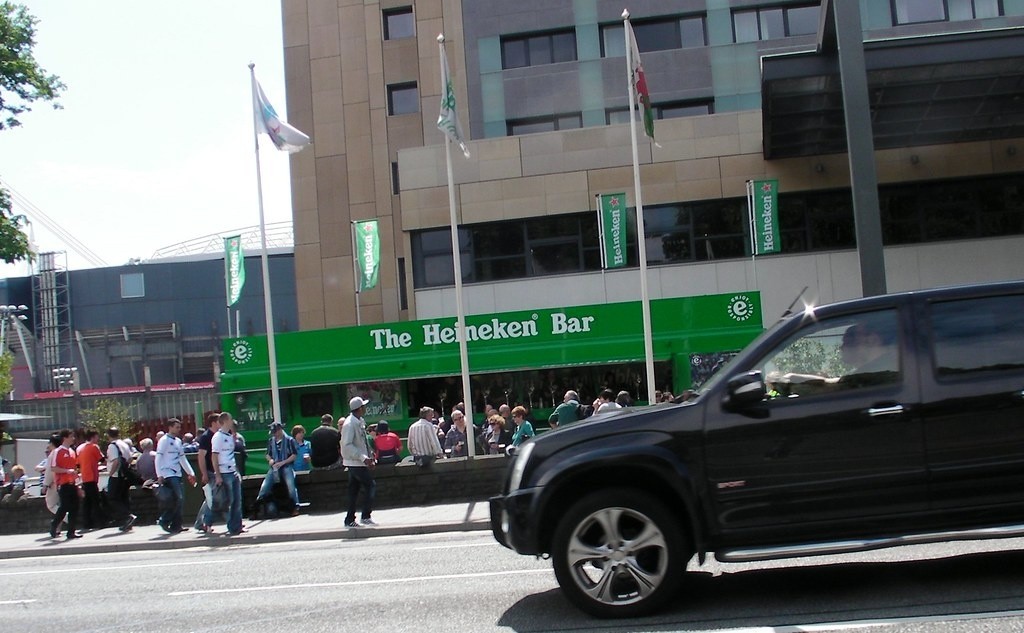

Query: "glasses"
<box><xmin>489</xmin><ymin>422</ymin><xmax>497</xmax><ymax>426</ymax></box>
<box><xmin>45</xmin><ymin>450</ymin><xmax>52</xmax><ymax>453</ymax></box>
<box><xmin>454</xmin><ymin>416</ymin><xmax>464</xmax><ymax>421</ymax></box>
<box><xmin>512</xmin><ymin>415</ymin><xmax>517</xmax><ymax>418</ymax></box>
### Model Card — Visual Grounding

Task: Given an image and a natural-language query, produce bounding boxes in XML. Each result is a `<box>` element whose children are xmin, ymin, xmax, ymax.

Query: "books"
<box><xmin>628</xmin><ymin>23</ymin><xmax>663</xmax><ymax>148</ymax></box>
<box><xmin>437</xmin><ymin>43</ymin><xmax>471</xmax><ymax>158</ymax></box>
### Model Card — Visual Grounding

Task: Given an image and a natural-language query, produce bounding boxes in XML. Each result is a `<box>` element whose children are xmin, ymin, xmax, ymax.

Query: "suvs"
<box><xmin>487</xmin><ymin>277</ymin><xmax>1024</xmax><ymax>620</ymax></box>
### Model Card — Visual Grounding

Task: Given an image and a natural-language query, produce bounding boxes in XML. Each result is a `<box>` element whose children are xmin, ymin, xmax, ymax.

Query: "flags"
<box><xmin>251</xmin><ymin>68</ymin><xmax>311</xmax><ymax>155</ymax></box>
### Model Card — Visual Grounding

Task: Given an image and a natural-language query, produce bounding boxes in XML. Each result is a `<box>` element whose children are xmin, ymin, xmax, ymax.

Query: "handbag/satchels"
<box><xmin>157</xmin><ymin>482</ymin><xmax>176</xmax><ymax>510</ymax></box>
<box><xmin>119</xmin><ymin>458</ymin><xmax>133</xmax><ymax>480</ymax></box>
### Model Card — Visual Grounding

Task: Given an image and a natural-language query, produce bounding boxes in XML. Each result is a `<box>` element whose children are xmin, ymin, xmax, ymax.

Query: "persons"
<box><xmin>764</xmin><ymin>325</ymin><xmax>890</xmax><ymax>396</ymax></box>
<box><xmin>107</xmin><ymin>427</ymin><xmax>137</xmax><ymax>532</ymax></box>
<box><xmin>548</xmin><ymin>390</ymin><xmax>633</xmax><ymax>429</ymax></box>
<box><xmin>249</xmin><ymin>420</ymin><xmax>311</xmax><ymax>518</ymax></box>
<box><xmin>136</xmin><ymin>438</ymin><xmax>157</xmax><ymax>482</ymax></box>
<box><xmin>155</xmin><ymin>413</ymin><xmax>249</xmax><ymax>533</ymax></box>
<box><xmin>77</xmin><ymin>430</ymin><xmax>107</xmax><ymax>533</ymax></box>
<box><xmin>655</xmin><ymin>390</ymin><xmax>675</xmax><ymax>403</ymax></box>
<box><xmin>340</xmin><ymin>396</ymin><xmax>379</xmax><ymax>529</ymax></box>
<box><xmin>0</xmin><ymin>458</ymin><xmax>9</xmax><ymax>482</ymax></box>
<box><xmin>35</xmin><ymin>428</ymin><xmax>83</xmax><ymax>538</ymax></box>
<box><xmin>444</xmin><ymin>403</ymin><xmax>535</xmax><ymax>457</ymax></box>
<box><xmin>11</xmin><ymin>465</ymin><xmax>27</xmax><ymax>490</ymax></box>
<box><xmin>289</xmin><ymin>406</ymin><xmax>444</xmax><ymax>471</ymax></box>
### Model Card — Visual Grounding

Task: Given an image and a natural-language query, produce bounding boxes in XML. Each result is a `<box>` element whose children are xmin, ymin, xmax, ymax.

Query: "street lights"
<box><xmin>0</xmin><ymin>305</ymin><xmax>28</xmax><ymax>353</ymax></box>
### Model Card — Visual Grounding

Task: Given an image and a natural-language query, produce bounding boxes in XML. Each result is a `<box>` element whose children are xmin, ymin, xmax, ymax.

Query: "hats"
<box><xmin>349</xmin><ymin>396</ymin><xmax>369</xmax><ymax>411</ymax></box>
<box><xmin>268</xmin><ymin>422</ymin><xmax>282</xmax><ymax>434</ymax></box>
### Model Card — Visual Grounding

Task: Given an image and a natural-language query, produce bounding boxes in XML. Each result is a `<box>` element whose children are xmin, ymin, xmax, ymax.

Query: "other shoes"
<box><xmin>159</xmin><ymin>519</ymin><xmax>169</xmax><ymax>531</ymax></box>
<box><xmin>119</xmin><ymin>514</ymin><xmax>136</xmax><ymax>531</ymax></box>
<box><xmin>249</xmin><ymin>514</ymin><xmax>255</xmax><ymax>520</ymax></box>
<box><xmin>292</xmin><ymin>510</ymin><xmax>300</xmax><ymax>516</ymax></box>
<box><xmin>50</xmin><ymin>520</ymin><xmax>57</xmax><ymax>538</ymax></box>
<box><xmin>345</xmin><ymin>522</ymin><xmax>359</xmax><ymax>527</ymax></box>
<box><xmin>66</xmin><ymin>532</ymin><xmax>82</xmax><ymax>539</ymax></box>
<box><xmin>360</xmin><ymin>519</ymin><xmax>377</xmax><ymax>525</ymax></box>
<box><xmin>200</xmin><ymin>525</ymin><xmax>213</xmax><ymax>532</ymax></box>
<box><xmin>240</xmin><ymin>528</ymin><xmax>247</xmax><ymax>533</ymax></box>
<box><xmin>172</xmin><ymin>527</ymin><xmax>188</xmax><ymax>531</ymax></box>
